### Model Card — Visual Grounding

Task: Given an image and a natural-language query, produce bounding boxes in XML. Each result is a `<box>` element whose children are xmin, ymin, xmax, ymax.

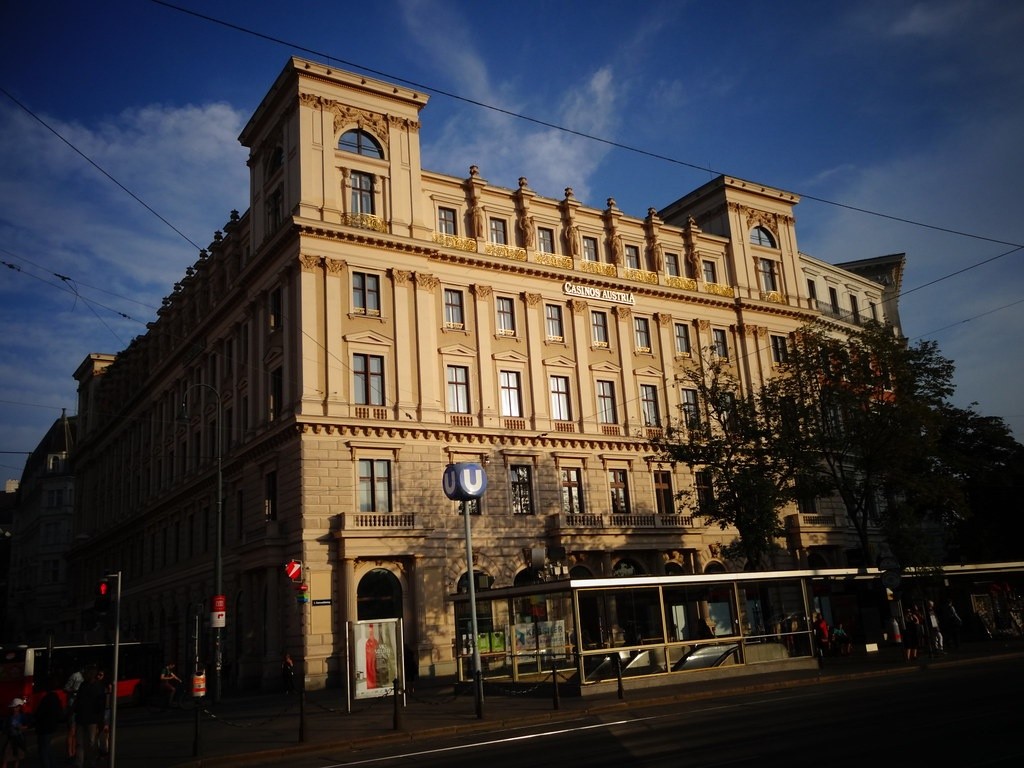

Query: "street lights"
<box><xmin>179</xmin><ymin>379</ymin><xmax>223</xmax><ymax>711</ymax></box>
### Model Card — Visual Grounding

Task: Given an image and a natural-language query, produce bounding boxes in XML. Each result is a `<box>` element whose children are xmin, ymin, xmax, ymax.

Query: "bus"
<box><xmin>0</xmin><ymin>641</ymin><xmax>164</xmax><ymax>723</ymax></box>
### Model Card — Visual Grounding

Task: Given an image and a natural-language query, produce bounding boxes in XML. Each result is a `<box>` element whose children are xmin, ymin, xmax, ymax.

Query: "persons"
<box><xmin>0</xmin><ymin>663</ymin><xmax>110</xmax><ymax>768</ymax></box>
<box><xmin>160</xmin><ymin>663</ymin><xmax>184</xmax><ymax>707</ymax></box>
<box><xmin>898</xmin><ymin>598</ymin><xmax>945</xmax><ymax>664</ymax></box>
<box><xmin>773</xmin><ymin>609</ymin><xmax>856</xmax><ymax>662</ymax></box>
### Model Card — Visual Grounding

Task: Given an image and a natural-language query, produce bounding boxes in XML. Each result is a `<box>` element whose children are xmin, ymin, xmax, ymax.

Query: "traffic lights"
<box><xmin>93</xmin><ymin>578</ymin><xmax>110</xmax><ymax>595</ymax></box>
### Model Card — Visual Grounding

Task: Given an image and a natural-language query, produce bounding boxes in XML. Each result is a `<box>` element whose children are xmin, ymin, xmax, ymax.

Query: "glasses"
<box><xmin>906</xmin><ymin>610</ymin><xmax>913</xmax><ymax>613</ymax></box>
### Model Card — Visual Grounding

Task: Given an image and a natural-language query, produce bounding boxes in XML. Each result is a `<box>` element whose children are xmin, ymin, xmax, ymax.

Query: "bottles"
<box><xmin>375</xmin><ymin>623</ymin><xmax>384</xmax><ymax>687</ymax></box>
<box><xmin>366</xmin><ymin>624</ymin><xmax>377</xmax><ymax>689</ymax></box>
<box><xmin>384</xmin><ymin>623</ymin><xmax>395</xmax><ymax>687</ymax></box>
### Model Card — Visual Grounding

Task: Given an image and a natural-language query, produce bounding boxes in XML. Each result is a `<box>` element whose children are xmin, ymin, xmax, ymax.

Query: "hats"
<box><xmin>8</xmin><ymin>698</ymin><xmax>24</xmax><ymax>708</ymax></box>
